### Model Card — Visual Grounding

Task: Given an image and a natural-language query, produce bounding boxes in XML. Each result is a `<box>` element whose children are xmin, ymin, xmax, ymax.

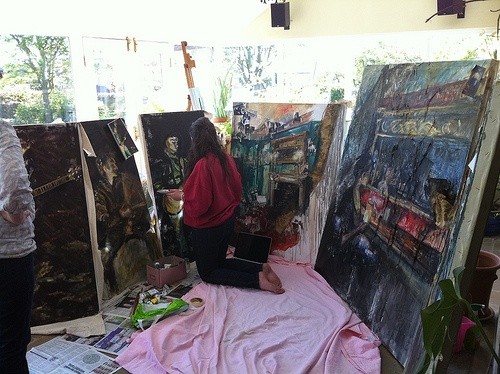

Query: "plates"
<box><xmin>159</xmin><ymin>190</ymin><xmax>181</xmax><ymax>192</ymax></box>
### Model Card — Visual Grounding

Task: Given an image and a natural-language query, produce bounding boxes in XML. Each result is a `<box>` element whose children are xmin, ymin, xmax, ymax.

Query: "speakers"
<box><xmin>437</xmin><ymin>0</ymin><xmax>466</xmax><ymax>16</ymax></box>
<box><xmin>271</xmin><ymin>2</ymin><xmax>290</xmax><ymax>30</ymax></box>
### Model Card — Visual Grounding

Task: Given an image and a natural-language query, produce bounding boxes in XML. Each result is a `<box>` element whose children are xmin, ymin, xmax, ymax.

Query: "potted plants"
<box><xmin>212</xmin><ymin>75</ymin><xmax>228</xmax><ymax>123</ymax></box>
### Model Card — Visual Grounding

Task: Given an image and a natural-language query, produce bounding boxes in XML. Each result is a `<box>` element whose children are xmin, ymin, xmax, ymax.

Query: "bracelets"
<box><xmin>180</xmin><ymin>192</ymin><xmax>184</xmax><ymax>201</ymax></box>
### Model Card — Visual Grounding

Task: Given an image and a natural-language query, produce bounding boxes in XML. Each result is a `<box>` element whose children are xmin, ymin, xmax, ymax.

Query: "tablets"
<box><xmin>234</xmin><ymin>231</ymin><xmax>272</xmax><ymax>263</ymax></box>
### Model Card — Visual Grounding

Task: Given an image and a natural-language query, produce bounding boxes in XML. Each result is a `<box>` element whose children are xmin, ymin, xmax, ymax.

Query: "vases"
<box><xmin>469</xmin><ymin>250</ymin><xmax>500</xmax><ymax>322</ymax></box>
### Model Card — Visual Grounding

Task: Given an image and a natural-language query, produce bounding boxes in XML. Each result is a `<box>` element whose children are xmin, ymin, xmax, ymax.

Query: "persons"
<box><xmin>0</xmin><ymin>119</ymin><xmax>35</xmax><ymax>374</ymax></box>
<box><xmin>157</xmin><ymin>117</ymin><xmax>285</xmax><ymax>294</ymax></box>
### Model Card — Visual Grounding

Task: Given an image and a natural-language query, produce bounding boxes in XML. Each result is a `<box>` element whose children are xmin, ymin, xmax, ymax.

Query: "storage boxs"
<box><xmin>145</xmin><ymin>256</ymin><xmax>186</xmax><ymax>287</ymax></box>
